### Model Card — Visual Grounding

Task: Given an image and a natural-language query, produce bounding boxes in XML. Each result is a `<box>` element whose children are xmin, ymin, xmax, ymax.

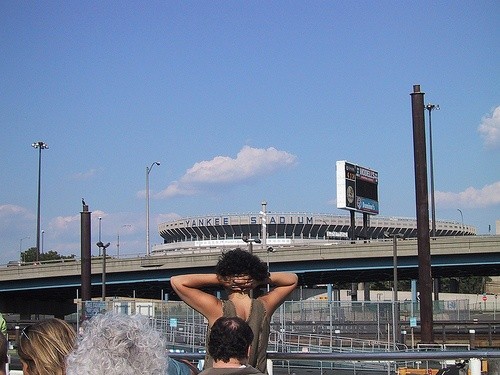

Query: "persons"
<box><xmin>0</xmin><ymin>329</ymin><xmax>9</xmax><ymax>375</ymax></box>
<box><xmin>63</xmin><ymin>310</ymin><xmax>169</xmax><ymax>375</ymax></box>
<box><xmin>194</xmin><ymin>316</ymin><xmax>264</xmax><ymax>375</ymax></box>
<box><xmin>170</xmin><ymin>248</ymin><xmax>299</xmax><ymax>375</ymax></box>
<box><xmin>17</xmin><ymin>318</ymin><xmax>78</xmax><ymax>375</ymax></box>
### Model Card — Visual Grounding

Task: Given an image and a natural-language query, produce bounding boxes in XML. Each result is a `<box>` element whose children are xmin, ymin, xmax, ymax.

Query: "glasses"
<box><xmin>21</xmin><ymin>324</ymin><xmax>33</xmax><ymax>341</ymax></box>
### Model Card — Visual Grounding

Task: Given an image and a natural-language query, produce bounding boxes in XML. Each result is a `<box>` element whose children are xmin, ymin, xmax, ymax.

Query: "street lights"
<box><xmin>456</xmin><ymin>208</ymin><xmax>465</xmax><ymax>234</ymax></box>
<box><xmin>19</xmin><ymin>236</ymin><xmax>31</xmax><ymax>265</ymax></box>
<box><xmin>145</xmin><ymin>160</ymin><xmax>164</xmax><ymax>254</ymax></box>
<box><xmin>31</xmin><ymin>140</ymin><xmax>45</xmax><ymax>260</ymax></box>
<box><xmin>117</xmin><ymin>224</ymin><xmax>131</xmax><ymax>256</ymax></box>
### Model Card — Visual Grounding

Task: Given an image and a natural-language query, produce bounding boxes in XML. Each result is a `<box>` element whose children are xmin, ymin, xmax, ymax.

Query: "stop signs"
<box><xmin>482</xmin><ymin>295</ymin><xmax>486</xmax><ymax>302</ymax></box>
